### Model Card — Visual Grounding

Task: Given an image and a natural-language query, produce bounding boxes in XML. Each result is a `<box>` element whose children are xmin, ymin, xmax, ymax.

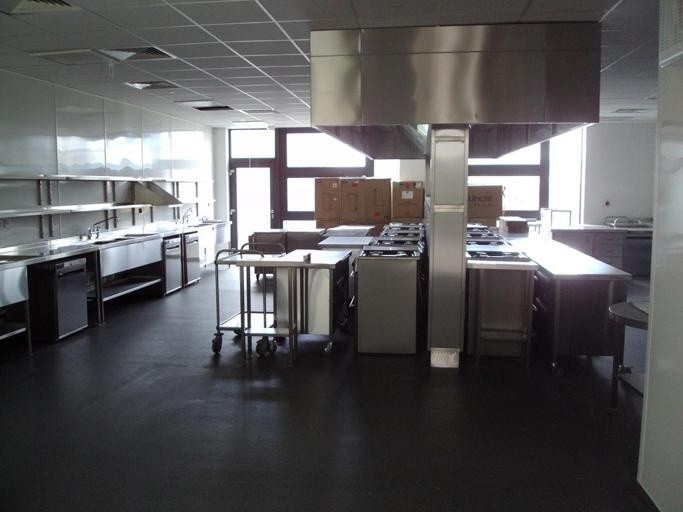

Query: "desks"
<box><xmin>607</xmin><ymin>300</ymin><xmax>649</xmax><ymax>408</ymax></box>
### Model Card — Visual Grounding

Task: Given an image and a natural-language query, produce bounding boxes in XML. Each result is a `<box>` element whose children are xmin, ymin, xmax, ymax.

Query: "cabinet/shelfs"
<box><xmin>553</xmin><ymin>232</ymin><xmax>652</xmax><ymax>279</ymax></box>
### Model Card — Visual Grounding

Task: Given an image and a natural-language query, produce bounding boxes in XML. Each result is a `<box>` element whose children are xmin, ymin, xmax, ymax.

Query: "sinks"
<box><xmin>188</xmin><ymin>224</ymin><xmax>211</xmax><ymax>228</ymax></box>
<box><xmin>0</xmin><ymin>255</ymin><xmax>43</xmax><ymax>266</ymax></box>
<box><xmin>87</xmin><ymin>238</ymin><xmax>134</xmax><ymax>245</ymax></box>
<box><xmin>203</xmin><ymin>220</ymin><xmax>227</xmax><ymax>223</ymax></box>
<box><xmin>120</xmin><ymin>234</ymin><xmax>157</xmax><ymax>238</ymax></box>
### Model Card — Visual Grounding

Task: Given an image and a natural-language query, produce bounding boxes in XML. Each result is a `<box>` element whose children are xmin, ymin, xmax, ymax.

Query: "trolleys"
<box><xmin>211</xmin><ymin>242</ymin><xmax>283</xmax><ymax>356</ymax></box>
<box><xmin>493</xmin><ymin>226</ymin><xmax>630</xmax><ymax>378</ymax></box>
<box><xmin>267</xmin><ymin>248</ymin><xmax>348</xmax><ymax>354</ymax></box>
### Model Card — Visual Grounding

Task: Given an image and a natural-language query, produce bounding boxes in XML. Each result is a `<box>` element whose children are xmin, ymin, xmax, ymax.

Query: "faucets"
<box><xmin>91</xmin><ymin>216</ymin><xmax>119</xmax><ymax>233</ymax></box>
<box><xmin>202</xmin><ymin>216</ymin><xmax>207</xmax><ymax>223</ymax></box>
<box><xmin>183</xmin><ymin>207</ymin><xmax>193</xmax><ymax>225</ymax></box>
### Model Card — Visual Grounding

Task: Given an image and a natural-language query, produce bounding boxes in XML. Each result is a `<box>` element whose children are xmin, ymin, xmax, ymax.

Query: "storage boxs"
<box><xmin>468</xmin><ymin>185</ymin><xmax>502</xmax><ymax>226</ymax></box>
<box><xmin>314</xmin><ymin>178</ymin><xmax>424</xmax><ymax>229</ymax></box>
